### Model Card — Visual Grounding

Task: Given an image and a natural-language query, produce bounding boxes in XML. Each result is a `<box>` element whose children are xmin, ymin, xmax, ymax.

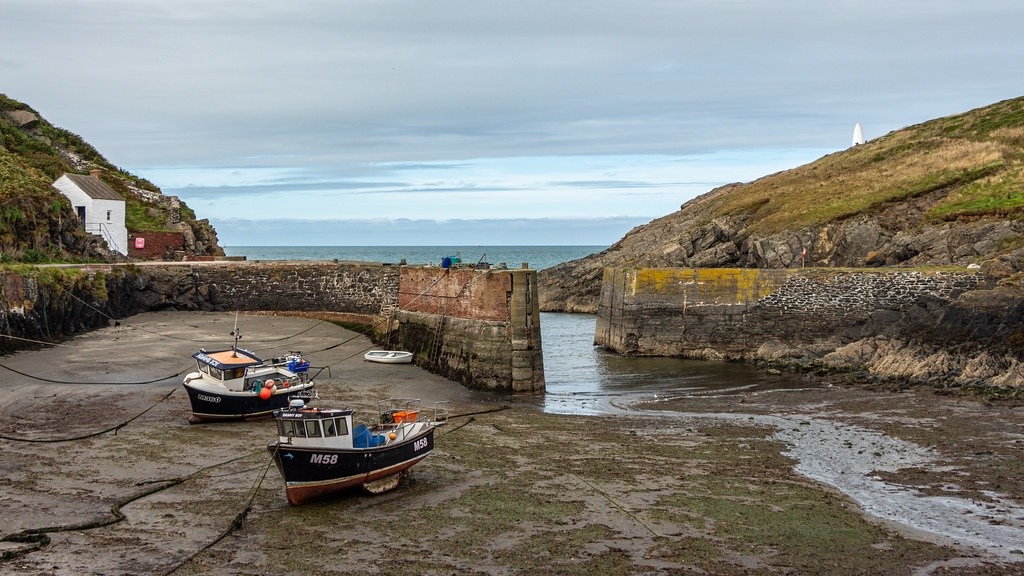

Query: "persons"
<box><xmin>77</xmin><ymin>213</ymin><xmax>84</xmax><ymax>230</ymax></box>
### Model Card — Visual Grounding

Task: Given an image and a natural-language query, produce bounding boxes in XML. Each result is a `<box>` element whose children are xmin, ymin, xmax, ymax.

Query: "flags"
<box><xmin>803</xmin><ymin>247</ymin><xmax>807</xmax><ymax>257</ymax></box>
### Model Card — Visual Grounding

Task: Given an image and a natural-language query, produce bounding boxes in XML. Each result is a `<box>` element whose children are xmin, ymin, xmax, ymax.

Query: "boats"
<box><xmin>266</xmin><ymin>377</ymin><xmax>449</xmax><ymax>505</ymax></box>
<box><xmin>364</xmin><ymin>350</ymin><xmax>413</xmax><ymax>364</ymax></box>
<box><xmin>182</xmin><ymin>311</ymin><xmax>320</xmax><ymax>424</ymax></box>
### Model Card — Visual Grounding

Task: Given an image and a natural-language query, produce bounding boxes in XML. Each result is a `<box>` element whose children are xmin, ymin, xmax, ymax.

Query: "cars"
<box><xmin>967</xmin><ymin>264</ymin><xmax>980</xmax><ymax>268</ymax></box>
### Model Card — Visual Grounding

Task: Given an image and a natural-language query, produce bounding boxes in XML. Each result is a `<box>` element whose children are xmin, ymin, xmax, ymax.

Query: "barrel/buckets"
<box><xmin>352</xmin><ymin>424</ymin><xmax>372</xmax><ymax>448</ymax></box>
<box><xmin>441</xmin><ymin>256</ymin><xmax>461</xmax><ymax>268</ymax></box>
